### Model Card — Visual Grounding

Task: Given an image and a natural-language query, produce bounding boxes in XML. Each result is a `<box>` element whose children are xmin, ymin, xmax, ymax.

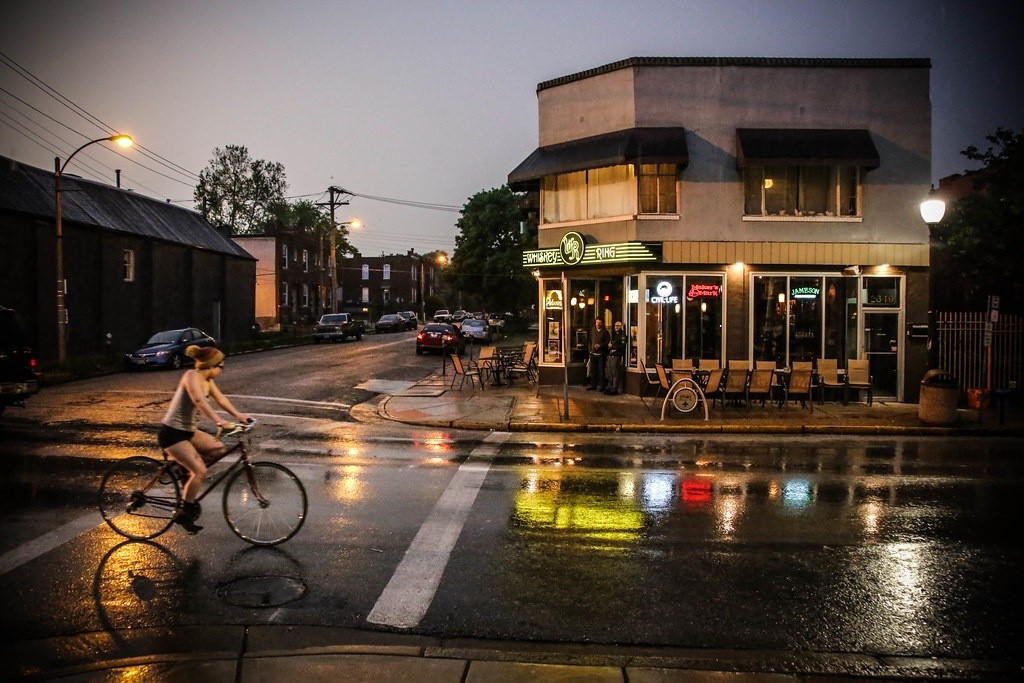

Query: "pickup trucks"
<box><xmin>312</xmin><ymin>313</ymin><xmax>365</xmax><ymax>342</ymax></box>
<box><xmin>433</xmin><ymin>310</ymin><xmax>452</xmax><ymax>324</ymax></box>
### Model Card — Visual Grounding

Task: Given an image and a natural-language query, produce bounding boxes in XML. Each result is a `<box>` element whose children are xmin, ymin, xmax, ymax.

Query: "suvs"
<box><xmin>452</xmin><ymin>310</ymin><xmax>467</xmax><ymax>323</ymax></box>
<box><xmin>0</xmin><ymin>304</ymin><xmax>40</xmax><ymax>414</ymax></box>
<box><xmin>487</xmin><ymin>312</ymin><xmax>506</xmax><ymax>327</ymax></box>
<box><xmin>396</xmin><ymin>311</ymin><xmax>418</xmax><ymax>331</ymax></box>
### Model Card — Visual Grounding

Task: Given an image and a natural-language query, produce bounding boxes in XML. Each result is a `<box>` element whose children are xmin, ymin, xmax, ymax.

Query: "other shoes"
<box><xmin>587</xmin><ymin>387</ymin><xmax>597</xmax><ymax>390</ymax></box>
<box><xmin>600</xmin><ymin>387</ymin><xmax>606</xmax><ymax>391</ymax></box>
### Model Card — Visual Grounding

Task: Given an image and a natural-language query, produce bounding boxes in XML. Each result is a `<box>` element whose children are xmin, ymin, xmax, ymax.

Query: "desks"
<box><xmin>479</xmin><ymin>357</ymin><xmax>515</xmax><ymax>386</ymax></box>
<box><xmin>493</xmin><ymin>352</ymin><xmax>526</xmax><ymax>355</ymax></box>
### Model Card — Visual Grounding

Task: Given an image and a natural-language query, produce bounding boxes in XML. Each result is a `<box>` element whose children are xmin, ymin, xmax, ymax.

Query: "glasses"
<box><xmin>216</xmin><ymin>364</ymin><xmax>225</xmax><ymax>369</ymax></box>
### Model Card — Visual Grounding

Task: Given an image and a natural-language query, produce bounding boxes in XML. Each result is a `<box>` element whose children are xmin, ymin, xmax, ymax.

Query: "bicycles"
<box><xmin>97</xmin><ymin>418</ymin><xmax>309</xmax><ymax>546</ymax></box>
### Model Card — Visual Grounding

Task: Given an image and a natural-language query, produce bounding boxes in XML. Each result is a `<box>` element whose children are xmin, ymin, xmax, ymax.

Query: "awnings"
<box><xmin>508</xmin><ymin>127</ymin><xmax>689</xmax><ymax>192</ymax></box>
<box><xmin>517</xmin><ymin>191</ymin><xmax>540</xmax><ymax>215</ymax></box>
<box><xmin>736</xmin><ymin>127</ymin><xmax>880</xmax><ymax>173</ymax></box>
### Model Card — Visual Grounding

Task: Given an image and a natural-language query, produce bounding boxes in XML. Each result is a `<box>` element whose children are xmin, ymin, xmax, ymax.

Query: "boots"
<box><xmin>172</xmin><ymin>500</ymin><xmax>204</xmax><ymax>531</ymax></box>
<box><xmin>175</xmin><ymin>466</ymin><xmax>189</xmax><ymax>483</ymax></box>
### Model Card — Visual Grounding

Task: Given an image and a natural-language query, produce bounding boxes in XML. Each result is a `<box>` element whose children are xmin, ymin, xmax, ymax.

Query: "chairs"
<box><xmin>639</xmin><ymin>359</ymin><xmax>873</xmax><ymax>414</ymax></box>
<box><xmin>451</xmin><ymin>342</ymin><xmax>539</xmax><ymax>393</ymax></box>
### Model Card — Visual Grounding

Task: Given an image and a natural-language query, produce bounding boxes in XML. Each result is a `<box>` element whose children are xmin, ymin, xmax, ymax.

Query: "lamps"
<box><xmin>844</xmin><ymin>266</ymin><xmax>860</xmax><ymax>275</ymax></box>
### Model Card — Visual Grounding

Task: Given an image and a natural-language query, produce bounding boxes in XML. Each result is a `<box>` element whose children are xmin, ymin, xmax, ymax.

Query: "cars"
<box><xmin>416</xmin><ymin>323</ymin><xmax>465</xmax><ymax>355</ymax></box>
<box><xmin>458</xmin><ymin>319</ymin><xmax>492</xmax><ymax>344</ymax></box>
<box><xmin>466</xmin><ymin>312</ymin><xmax>473</xmax><ymax>319</ymax></box>
<box><xmin>473</xmin><ymin>312</ymin><xmax>482</xmax><ymax>320</ymax></box>
<box><xmin>375</xmin><ymin>314</ymin><xmax>406</xmax><ymax>333</ymax></box>
<box><xmin>124</xmin><ymin>328</ymin><xmax>215</xmax><ymax>370</ymax></box>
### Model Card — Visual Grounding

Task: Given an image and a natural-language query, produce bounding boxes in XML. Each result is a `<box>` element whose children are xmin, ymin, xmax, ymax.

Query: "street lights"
<box><xmin>320</xmin><ymin>221</ymin><xmax>361</xmax><ymax>315</ymax></box>
<box><xmin>55</xmin><ymin>135</ymin><xmax>132</xmax><ymax>363</ymax></box>
<box><xmin>919</xmin><ymin>183</ymin><xmax>946</xmax><ymax>382</ymax></box>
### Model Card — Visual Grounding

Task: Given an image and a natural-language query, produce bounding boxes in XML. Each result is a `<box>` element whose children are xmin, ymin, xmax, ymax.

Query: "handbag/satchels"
<box><xmin>586</xmin><ymin>357</ymin><xmax>593</xmax><ymax>377</ymax></box>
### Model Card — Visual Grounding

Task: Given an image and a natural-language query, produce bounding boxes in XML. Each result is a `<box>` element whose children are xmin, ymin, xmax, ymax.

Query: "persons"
<box><xmin>158</xmin><ymin>344</ymin><xmax>247</xmax><ymax>532</ymax></box>
<box><xmin>586</xmin><ymin>317</ymin><xmax>610</xmax><ymax>392</ymax></box>
<box><xmin>603</xmin><ymin>321</ymin><xmax>627</xmax><ymax>396</ymax></box>
